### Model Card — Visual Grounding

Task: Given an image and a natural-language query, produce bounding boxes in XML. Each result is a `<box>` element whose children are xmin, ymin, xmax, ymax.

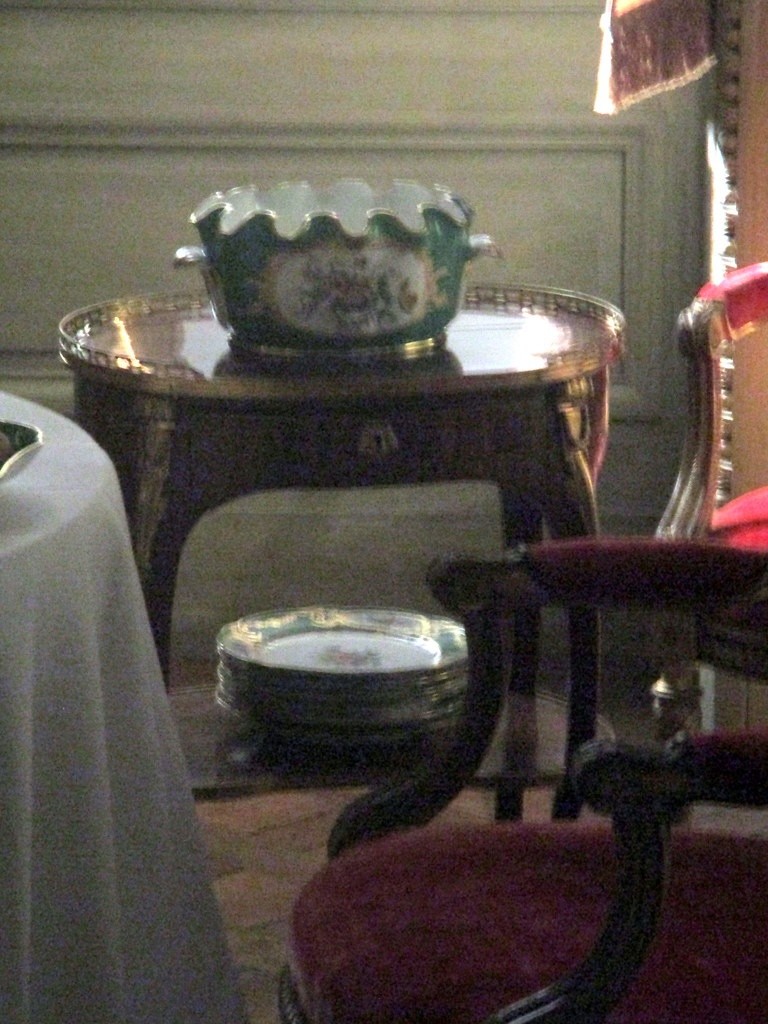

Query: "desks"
<box><xmin>1</xmin><ymin>384</ymin><xmax>255</xmax><ymax>1023</ymax></box>
<box><xmin>55</xmin><ymin>276</ymin><xmax>626</xmax><ymax>820</ymax></box>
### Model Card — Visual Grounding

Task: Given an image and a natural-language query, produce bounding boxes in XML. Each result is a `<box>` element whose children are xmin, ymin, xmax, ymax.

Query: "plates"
<box><xmin>214</xmin><ymin>604</ymin><xmax>468</xmax><ymax>742</ymax></box>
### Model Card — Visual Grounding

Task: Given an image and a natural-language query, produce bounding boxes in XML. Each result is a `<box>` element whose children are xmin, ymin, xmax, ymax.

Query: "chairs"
<box><xmin>271</xmin><ymin>537</ymin><xmax>768</xmax><ymax>1023</ymax></box>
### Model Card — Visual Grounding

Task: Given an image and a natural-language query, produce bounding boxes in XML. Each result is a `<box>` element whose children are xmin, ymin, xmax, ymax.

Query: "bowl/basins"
<box><xmin>186</xmin><ymin>180</ymin><xmax>473</xmax><ymax>360</ymax></box>
